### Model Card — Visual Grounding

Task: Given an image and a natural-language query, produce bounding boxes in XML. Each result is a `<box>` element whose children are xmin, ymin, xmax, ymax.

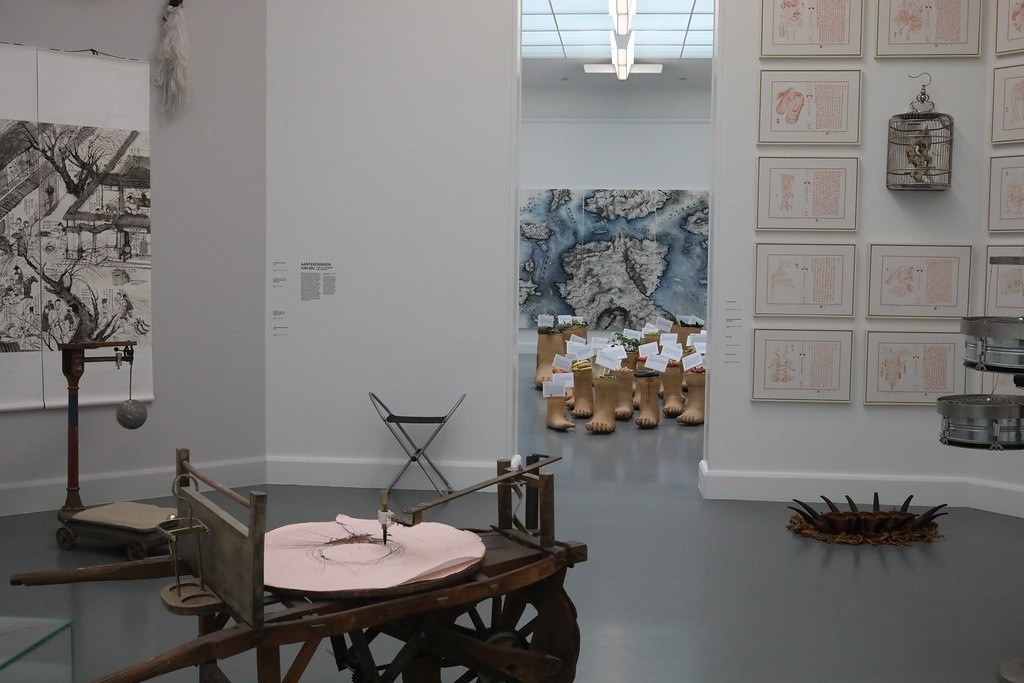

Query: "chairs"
<box><xmin>367</xmin><ymin>391</ymin><xmax>467</xmax><ymax>503</ymax></box>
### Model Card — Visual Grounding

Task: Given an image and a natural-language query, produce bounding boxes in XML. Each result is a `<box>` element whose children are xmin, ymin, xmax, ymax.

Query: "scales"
<box><xmin>55</xmin><ymin>340</ymin><xmax>177</xmax><ymax>563</ymax></box>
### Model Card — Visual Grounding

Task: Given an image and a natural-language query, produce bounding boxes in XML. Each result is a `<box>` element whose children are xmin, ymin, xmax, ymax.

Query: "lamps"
<box><xmin>609</xmin><ymin>0</ymin><xmax>636</xmax><ymax>81</ymax></box>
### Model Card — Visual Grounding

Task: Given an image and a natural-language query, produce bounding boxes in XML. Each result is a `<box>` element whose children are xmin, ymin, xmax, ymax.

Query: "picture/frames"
<box><xmin>991</xmin><ymin>63</ymin><xmax>1024</xmax><ymax>145</ymax></box>
<box><xmin>873</xmin><ymin>0</ymin><xmax>984</xmax><ymax>59</ymax></box>
<box><xmin>983</xmin><ymin>244</ymin><xmax>1024</xmax><ymax>317</ymax></box>
<box><xmin>863</xmin><ymin>330</ymin><xmax>968</xmax><ymax>408</ymax></box>
<box><xmin>994</xmin><ymin>0</ymin><xmax>1024</xmax><ymax>56</ymax></box>
<box><xmin>866</xmin><ymin>242</ymin><xmax>975</xmax><ymax>320</ymax></box>
<box><xmin>985</xmin><ymin>154</ymin><xmax>1024</xmax><ymax>233</ymax></box>
<box><xmin>981</xmin><ymin>369</ymin><xmax>1024</xmax><ymax>396</ymax></box>
<box><xmin>751</xmin><ymin>328</ymin><xmax>855</xmax><ymax>404</ymax></box>
<box><xmin>755</xmin><ymin>155</ymin><xmax>859</xmax><ymax>231</ymax></box>
<box><xmin>758</xmin><ymin>69</ymin><xmax>863</xmax><ymax>145</ymax></box>
<box><xmin>754</xmin><ymin>240</ymin><xmax>857</xmax><ymax>319</ymax></box>
<box><xmin>758</xmin><ymin>0</ymin><xmax>864</xmax><ymax>58</ymax></box>
